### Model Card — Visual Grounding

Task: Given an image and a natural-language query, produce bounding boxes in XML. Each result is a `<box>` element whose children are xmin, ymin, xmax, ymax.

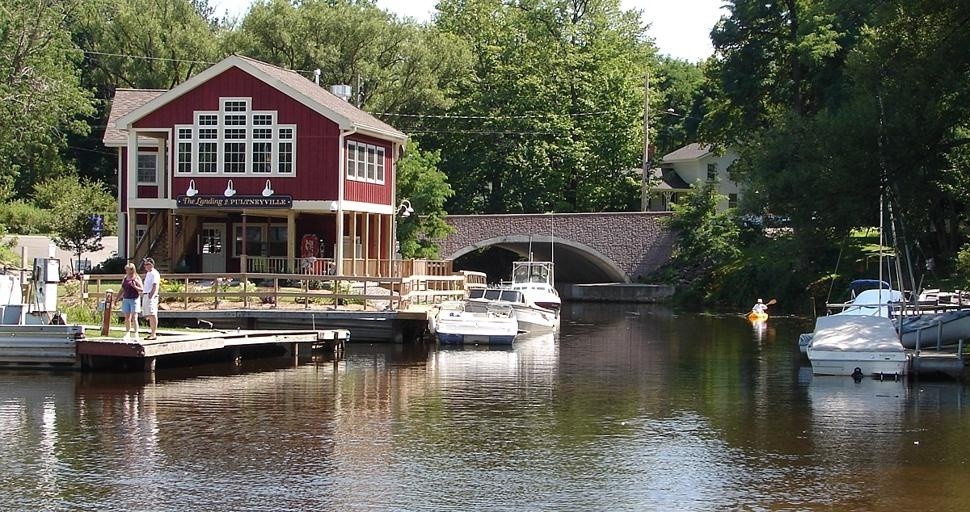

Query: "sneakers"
<box><xmin>121</xmin><ymin>335</ymin><xmax>157</xmax><ymax>342</ymax></box>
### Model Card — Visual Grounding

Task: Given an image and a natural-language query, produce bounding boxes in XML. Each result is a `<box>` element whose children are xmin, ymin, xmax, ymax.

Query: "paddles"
<box><xmin>746</xmin><ymin>299</ymin><xmax>777</xmax><ymax>316</ymax></box>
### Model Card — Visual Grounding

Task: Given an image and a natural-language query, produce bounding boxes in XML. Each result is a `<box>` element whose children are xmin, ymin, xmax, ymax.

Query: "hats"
<box><xmin>757</xmin><ymin>299</ymin><xmax>762</xmax><ymax>303</ymax></box>
<box><xmin>143</xmin><ymin>257</ymin><xmax>155</xmax><ymax>266</ymax></box>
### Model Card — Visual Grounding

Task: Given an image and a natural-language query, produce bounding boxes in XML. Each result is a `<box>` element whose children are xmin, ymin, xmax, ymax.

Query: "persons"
<box><xmin>114</xmin><ymin>263</ymin><xmax>143</xmax><ymax>342</ymax></box>
<box><xmin>142</xmin><ymin>257</ymin><xmax>160</xmax><ymax>340</ymax></box>
<box><xmin>752</xmin><ymin>298</ymin><xmax>768</xmax><ymax>313</ymax></box>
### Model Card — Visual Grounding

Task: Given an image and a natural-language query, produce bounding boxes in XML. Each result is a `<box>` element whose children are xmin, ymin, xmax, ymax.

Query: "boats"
<box><xmin>515</xmin><ymin>332</ymin><xmax>558</xmax><ymax>378</ymax></box>
<box><xmin>807</xmin><ymin>374</ymin><xmax>908</xmax><ymax>429</ymax></box>
<box><xmin>797</xmin><ymin>148</ymin><xmax>970</xmax><ymax>378</ymax></box>
<box><xmin>743</xmin><ymin>311</ymin><xmax>773</xmax><ymax>326</ymax></box>
<box><xmin>428</xmin><ymin>343</ymin><xmax>523</xmax><ymax>387</ymax></box>
<box><xmin>428</xmin><ymin>211</ymin><xmax>562</xmax><ymax>350</ymax></box>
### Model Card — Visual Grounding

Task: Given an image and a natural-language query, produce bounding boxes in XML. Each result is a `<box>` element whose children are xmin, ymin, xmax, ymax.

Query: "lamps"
<box><xmin>395</xmin><ymin>199</ymin><xmax>414</xmax><ymax>218</ymax></box>
<box><xmin>185</xmin><ymin>178</ymin><xmax>198</xmax><ymax>198</ymax></box>
<box><xmin>262</xmin><ymin>178</ymin><xmax>275</xmax><ymax>197</ymax></box>
<box><xmin>224</xmin><ymin>179</ymin><xmax>237</xmax><ymax>197</ymax></box>
